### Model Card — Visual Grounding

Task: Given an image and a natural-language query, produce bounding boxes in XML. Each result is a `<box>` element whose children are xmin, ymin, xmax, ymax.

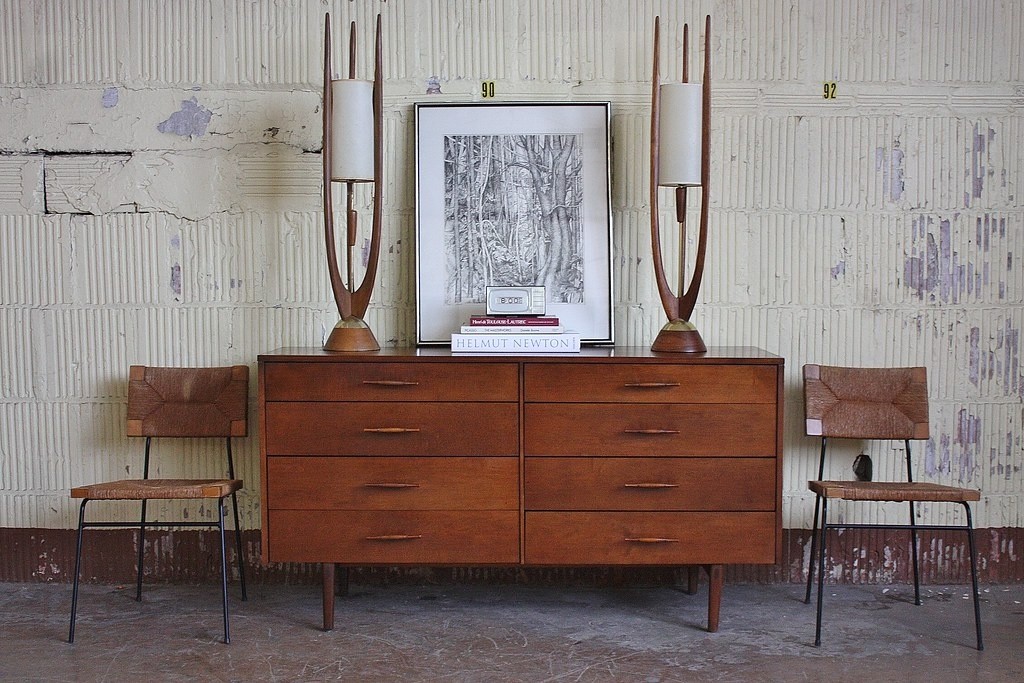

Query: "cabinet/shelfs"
<box><xmin>257</xmin><ymin>346</ymin><xmax>783</xmax><ymax>633</ymax></box>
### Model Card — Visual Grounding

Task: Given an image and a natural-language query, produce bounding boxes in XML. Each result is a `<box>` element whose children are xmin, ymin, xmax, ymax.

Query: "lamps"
<box><xmin>650</xmin><ymin>14</ymin><xmax>712</xmax><ymax>354</ymax></box>
<box><xmin>323</xmin><ymin>12</ymin><xmax>383</xmax><ymax>353</ymax></box>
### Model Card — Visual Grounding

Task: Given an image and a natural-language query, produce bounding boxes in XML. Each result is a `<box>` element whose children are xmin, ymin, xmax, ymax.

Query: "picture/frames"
<box><xmin>411</xmin><ymin>101</ymin><xmax>616</xmax><ymax>346</ymax></box>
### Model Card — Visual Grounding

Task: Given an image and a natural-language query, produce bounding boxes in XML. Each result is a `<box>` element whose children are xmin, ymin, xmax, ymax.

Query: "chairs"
<box><xmin>802</xmin><ymin>363</ymin><xmax>985</xmax><ymax>654</ymax></box>
<box><xmin>69</xmin><ymin>364</ymin><xmax>250</xmax><ymax>645</ymax></box>
